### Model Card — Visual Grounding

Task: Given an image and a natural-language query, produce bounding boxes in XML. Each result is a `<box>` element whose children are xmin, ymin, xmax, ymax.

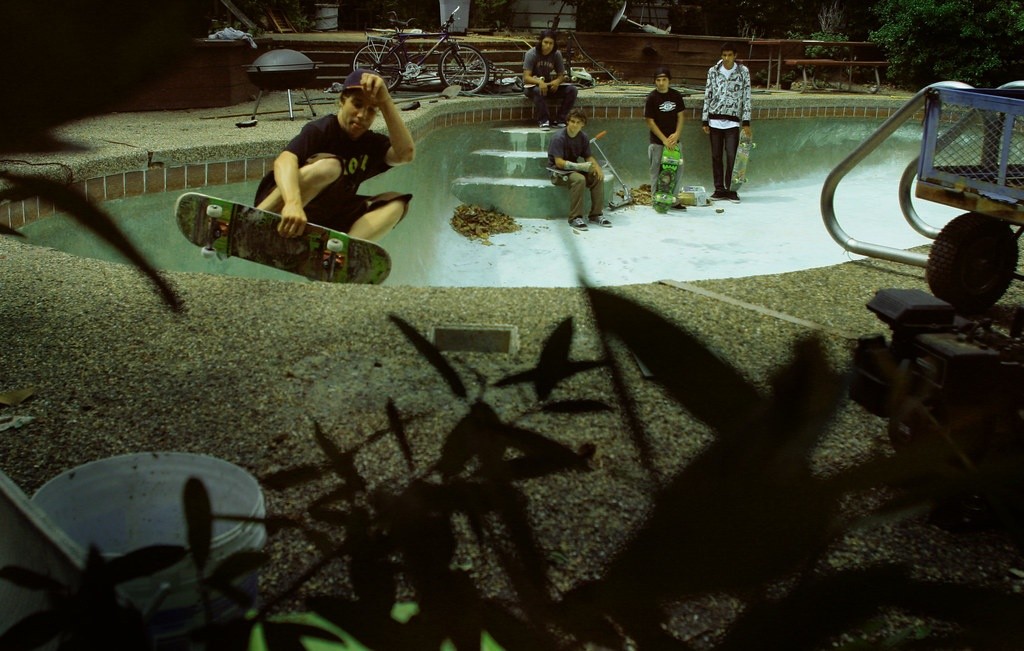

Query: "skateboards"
<box><xmin>729</xmin><ymin>129</ymin><xmax>756</xmax><ymax>192</ymax></box>
<box><xmin>653</xmin><ymin>143</ymin><xmax>680</xmax><ymax>214</ymax></box>
<box><xmin>174</xmin><ymin>192</ymin><xmax>392</xmax><ymax>286</ymax></box>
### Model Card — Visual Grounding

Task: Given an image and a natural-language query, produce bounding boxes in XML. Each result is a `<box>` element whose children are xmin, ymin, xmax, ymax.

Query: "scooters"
<box><xmin>588</xmin><ymin>130</ymin><xmax>637</xmax><ymax>209</ymax></box>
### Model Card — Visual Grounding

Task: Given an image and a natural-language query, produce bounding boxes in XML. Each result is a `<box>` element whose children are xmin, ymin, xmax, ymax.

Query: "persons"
<box><xmin>546</xmin><ymin>109</ymin><xmax>612</xmax><ymax>233</ymax></box>
<box><xmin>523</xmin><ymin>30</ymin><xmax>578</xmax><ymax>131</ymax></box>
<box><xmin>216</xmin><ymin>68</ymin><xmax>415</xmax><ymax>283</ymax></box>
<box><xmin>702</xmin><ymin>42</ymin><xmax>751</xmax><ymax>204</ymax></box>
<box><xmin>644</xmin><ymin>69</ymin><xmax>687</xmax><ymax>212</ymax></box>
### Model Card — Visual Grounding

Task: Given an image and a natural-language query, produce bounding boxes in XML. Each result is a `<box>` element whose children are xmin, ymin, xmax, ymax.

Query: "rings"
<box><xmin>673</xmin><ymin>142</ymin><xmax>675</xmax><ymax>144</ymax></box>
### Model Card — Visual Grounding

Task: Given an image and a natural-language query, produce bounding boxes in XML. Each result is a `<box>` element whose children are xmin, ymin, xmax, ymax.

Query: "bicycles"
<box><xmin>349</xmin><ymin>5</ymin><xmax>490</xmax><ymax>96</ymax></box>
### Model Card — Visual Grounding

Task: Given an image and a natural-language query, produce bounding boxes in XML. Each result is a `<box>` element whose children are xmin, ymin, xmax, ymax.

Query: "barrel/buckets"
<box><xmin>26</xmin><ymin>451</ymin><xmax>269</xmax><ymax>612</ymax></box>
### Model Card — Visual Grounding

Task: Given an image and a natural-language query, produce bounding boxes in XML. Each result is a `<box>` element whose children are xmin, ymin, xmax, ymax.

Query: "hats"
<box><xmin>342</xmin><ymin>69</ymin><xmax>388</xmax><ymax>91</ymax></box>
<box><xmin>652</xmin><ymin>67</ymin><xmax>671</xmax><ymax>84</ymax></box>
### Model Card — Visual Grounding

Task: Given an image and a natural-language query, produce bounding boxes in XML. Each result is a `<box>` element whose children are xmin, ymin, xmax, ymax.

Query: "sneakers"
<box><xmin>539</xmin><ymin>120</ymin><xmax>550</xmax><ymax>131</ymax></box>
<box><xmin>671</xmin><ymin>204</ymin><xmax>687</xmax><ymax>212</ymax></box>
<box><xmin>725</xmin><ymin>188</ymin><xmax>741</xmax><ymax>203</ymax></box>
<box><xmin>569</xmin><ymin>217</ymin><xmax>589</xmax><ymax>231</ymax></box>
<box><xmin>708</xmin><ymin>190</ymin><xmax>726</xmax><ymax>201</ymax></box>
<box><xmin>551</xmin><ymin>119</ymin><xmax>567</xmax><ymax>127</ymax></box>
<box><xmin>588</xmin><ymin>214</ymin><xmax>612</xmax><ymax>227</ymax></box>
